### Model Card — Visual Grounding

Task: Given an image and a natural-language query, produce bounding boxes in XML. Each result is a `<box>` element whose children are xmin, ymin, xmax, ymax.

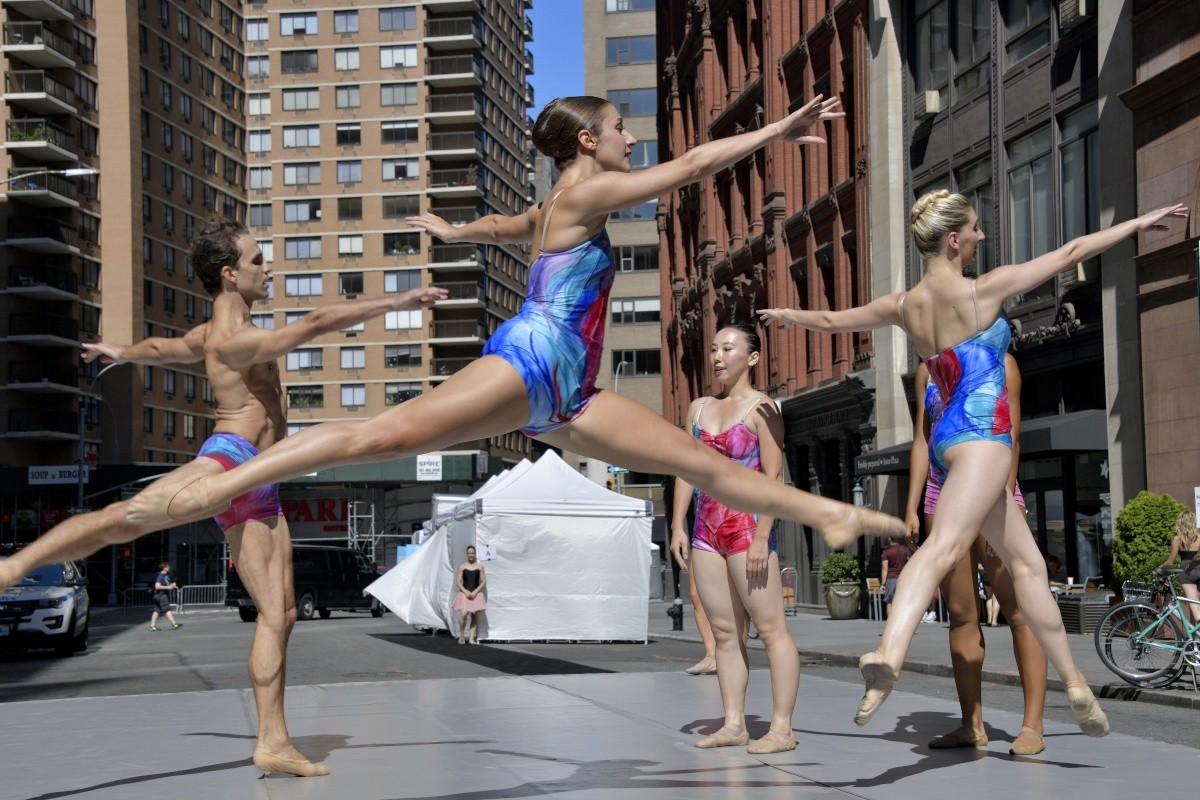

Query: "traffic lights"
<box><xmin>606</xmin><ymin>477</ymin><xmax>614</xmax><ymax>491</ymax></box>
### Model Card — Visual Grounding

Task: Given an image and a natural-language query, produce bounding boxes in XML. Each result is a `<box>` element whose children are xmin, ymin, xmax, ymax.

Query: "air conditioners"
<box><xmin>1058</xmin><ymin>256</ymin><xmax>1099</xmax><ymax>286</ymax></box>
<box><xmin>912</xmin><ymin>89</ymin><xmax>940</xmax><ymax>120</ymax></box>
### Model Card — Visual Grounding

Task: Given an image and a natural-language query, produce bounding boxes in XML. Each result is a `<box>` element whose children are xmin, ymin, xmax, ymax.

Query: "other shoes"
<box><xmin>1009</xmin><ymin>726</ymin><xmax>1045</xmax><ymax>755</ymax></box>
<box><xmin>823</xmin><ymin>503</ymin><xmax>906</xmax><ymax>550</ymax></box>
<box><xmin>695</xmin><ymin>726</ymin><xmax>750</xmax><ymax>747</ymax></box>
<box><xmin>126</xmin><ymin>456</ymin><xmax>225</xmax><ymax>527</ymax></box>
<box><xmin>0</xmin><ymin>560</ymin><xmax>23</xmax><ymax>592</ymax></box>
<box><xmin>172</xmin><ymin>623</ymin><xmax>183</xmax><ymax>630</ymax></box>
<box><xmin>1065</xmin><ymin>685</ymin><xmax>1111</xmax><ymax>737</ymax></box>
<box><xmin>149</xmin><ymin>626</ymin><xmax>161</xmax><ymax>631</ymax></box>
<box><xmin>854</xmin><ymin>651</ymin><xmax>899</xmax><ymax>727</ymax></box>
<box><xmin>747</xmin><ymin>733</ymin><xmax>796</xmax><ymax>754</ymax></box>
<box><xmin>929</xmin><ymin>725</ymin><xmax>988</xmax><ymax>749</ymax></box>
<box><xmin>685</xmin><ymin>656</ymin><xmax>716</xmax><ymax>675</ymax></box>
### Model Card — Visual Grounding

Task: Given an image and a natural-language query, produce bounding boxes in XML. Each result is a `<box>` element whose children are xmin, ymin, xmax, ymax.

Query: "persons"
<box><xmin>669</xmin><ymin>321</ymin><xmax>801</xmax><ymax>753</ymax></box>
<box><xmin>757</xmin><ymin>189</ymin><xmax>1188</xmax><ymax>737</ymax></box>
<box><xmin>451</xmin><ymin>546</ymin><xmax>487</xmax><ymax>645</ymax></box>
<box><xmin>1157</xmin><ymin>510</ymin><xmax>1200</xmax><ymax>627</ymax></box>
<box><xmin>685</xmin><ymin>531</ymin><xmax>751</xmax><ymax>674</ymax></box>
<box><xmin>0</xmin><ymin>220</ymin><xmax>447</xmax><ymax>778</ymax></box>
<box><xmin>902</xmin><ymin>272</ymin><xmax>1050</xmax><ymax>756</ymax></box>
<box><xmin>877</xmin><ymin>535</ymin><xmax>1069</xmax><ymax>628</ymax></box>
<box><xmin>148</xmin><ymin>562</ymin><xmax>184</xmax><ymax>631</ymax></box>
<box><xmin>124</xmin><ymin>92</ymin><xmax>912</xmax><ymax>553</ymax></box>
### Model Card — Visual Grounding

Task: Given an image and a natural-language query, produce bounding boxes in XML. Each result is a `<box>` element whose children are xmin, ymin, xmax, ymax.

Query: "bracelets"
<box><xmin>881</xmin><ymin>583</ymin><xmax>886</xmax><ymax>586</ymax></box>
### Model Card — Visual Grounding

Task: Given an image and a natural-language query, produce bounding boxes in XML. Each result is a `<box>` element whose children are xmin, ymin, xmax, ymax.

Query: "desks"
<box><xmin>1061</xmin><ymin>583</ymin><xmax>1104</xmax><ymax>596</ymax></box>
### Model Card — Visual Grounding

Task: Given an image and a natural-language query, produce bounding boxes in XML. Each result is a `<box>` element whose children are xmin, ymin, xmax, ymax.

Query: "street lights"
<box><xmin>615</xmin><ymin>360</ymin><xmax>628</xmax><ymax>495</ymax></box>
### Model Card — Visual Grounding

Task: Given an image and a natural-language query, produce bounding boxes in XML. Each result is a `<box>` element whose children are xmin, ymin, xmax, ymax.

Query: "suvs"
<box><xmin>0</xmin><ymin>543</ymin><xmax>90</xmax><ymax>659</ymax></box>
<box><xmin>223</xmin><ymin>544</ymin><xmax>390</xmax><ymax>624</ymax></box>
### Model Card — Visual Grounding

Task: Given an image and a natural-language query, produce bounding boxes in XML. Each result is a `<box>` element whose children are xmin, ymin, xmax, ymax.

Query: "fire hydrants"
<box><xmin>665</xmin><ymin>597</ymin><xmax>683</xmax><ymax>632</ymax></box>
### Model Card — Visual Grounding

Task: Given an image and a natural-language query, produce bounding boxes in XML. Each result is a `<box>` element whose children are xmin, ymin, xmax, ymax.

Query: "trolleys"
<box><xmin>780</xmin><ymin>567</ymin><xmax>798</xmax><ymax>616</ymax></box>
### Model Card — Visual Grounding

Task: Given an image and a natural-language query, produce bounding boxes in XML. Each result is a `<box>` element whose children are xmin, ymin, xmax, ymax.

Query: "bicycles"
<box><xmin>747</xmin><ymin>618</ymin><xmax>760</xmax><ymax>639</ymax></box>
<box><xmin>1093</xmin><ymin>567</ymin><xmax>1200</xmax><ymax>694</ymax></box>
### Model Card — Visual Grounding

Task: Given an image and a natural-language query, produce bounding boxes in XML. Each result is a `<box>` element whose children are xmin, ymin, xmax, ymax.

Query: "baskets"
<box><xmin>1122</xmin><ymin>579</ymin><xmax>1154</xmax><ymax>604</ymax></box>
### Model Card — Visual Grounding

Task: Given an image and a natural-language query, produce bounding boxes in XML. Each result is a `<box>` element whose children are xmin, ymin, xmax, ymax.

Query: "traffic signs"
<box><xmin>65</xmin><ymin>506</ymin><xmax>92</xmax><ymax>516</ymax></box>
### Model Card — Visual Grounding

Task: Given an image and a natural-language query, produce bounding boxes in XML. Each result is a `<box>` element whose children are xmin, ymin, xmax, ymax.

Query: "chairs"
<box><xmin>1084</xmin><ymin>576</ymin><xmax>1103</xmax><ymax>593</ymax></box>
<box><xmin>866</xmin><ymin>577</ymin><xmax>882</xmax><ymax>621</ymax></box>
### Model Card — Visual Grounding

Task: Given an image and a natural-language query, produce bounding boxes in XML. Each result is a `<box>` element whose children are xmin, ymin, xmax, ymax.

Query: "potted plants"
<box><xmin>821</xmin><ymin>553</ymin><xmax>862</xmax><ymax>619</ymax></box>
<box><xmin>1112</xmin><ymin>489</ymin><xmax>1193</xmax><ymax>638</ymax></box>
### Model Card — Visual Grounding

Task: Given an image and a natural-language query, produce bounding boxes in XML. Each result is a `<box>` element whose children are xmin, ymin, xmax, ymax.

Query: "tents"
<box><xmin>363</xmin><ymin>448</ymin><xmax>655</xmax><ymax>644</ymax></box>
